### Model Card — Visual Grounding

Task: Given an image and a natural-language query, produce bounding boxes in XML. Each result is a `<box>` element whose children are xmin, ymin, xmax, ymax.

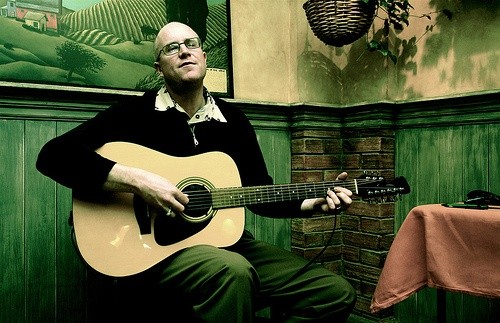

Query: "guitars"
<box><xmin>72</xmin><ymin>141</ymin><xmax>411</xmax><ymax>281</ymax></box>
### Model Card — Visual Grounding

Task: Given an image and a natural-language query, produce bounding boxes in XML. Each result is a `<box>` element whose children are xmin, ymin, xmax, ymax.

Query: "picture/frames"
<box><xmin>0</xmin><ymin>0</ymin><xmax>235</xmax><ymax>100</ymax></box>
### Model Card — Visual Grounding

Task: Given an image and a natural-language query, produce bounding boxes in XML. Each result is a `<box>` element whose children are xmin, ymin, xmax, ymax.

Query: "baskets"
<box><xmin>302</xmin><ymin>0</ymin><xmax>375</xmax><ymax>47</ymax></box>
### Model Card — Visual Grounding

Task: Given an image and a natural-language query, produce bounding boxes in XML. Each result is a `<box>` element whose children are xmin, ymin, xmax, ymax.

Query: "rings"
<box><xmin>166</xmin><ymin>210</ymin><xmax>171</xmax><ymax>215</ymax></box>
<box><xmin>336</xmin><ymin>206</ymin><xmax>341</xmax><ymax>209</ymax></box>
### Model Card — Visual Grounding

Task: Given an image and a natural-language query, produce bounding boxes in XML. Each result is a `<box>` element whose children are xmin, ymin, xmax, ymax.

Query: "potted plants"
<box><xmin>303</xmin><ymin>0</ymin><xmax>453</xmax><ymax>66</ymax></box>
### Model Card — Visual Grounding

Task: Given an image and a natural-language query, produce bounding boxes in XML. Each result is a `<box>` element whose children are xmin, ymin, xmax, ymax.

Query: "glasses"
<box><xmin>155</xmin><ymin>37</ymin><xmax>202</xmax><ymax>62</ymax></box>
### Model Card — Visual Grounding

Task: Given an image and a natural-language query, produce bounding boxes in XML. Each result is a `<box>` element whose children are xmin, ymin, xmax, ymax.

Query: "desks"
<box><xmin>414</xmin><ymin>202</ymin><xmax>500</xmax><ymax>323</ymax></box>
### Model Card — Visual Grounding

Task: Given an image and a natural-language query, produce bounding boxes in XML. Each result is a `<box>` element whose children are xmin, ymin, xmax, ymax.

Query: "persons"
<box><xmin>36</xmin><ymin>22</ymin><xmax>355</xmax><ymax>323</ymax></box>
